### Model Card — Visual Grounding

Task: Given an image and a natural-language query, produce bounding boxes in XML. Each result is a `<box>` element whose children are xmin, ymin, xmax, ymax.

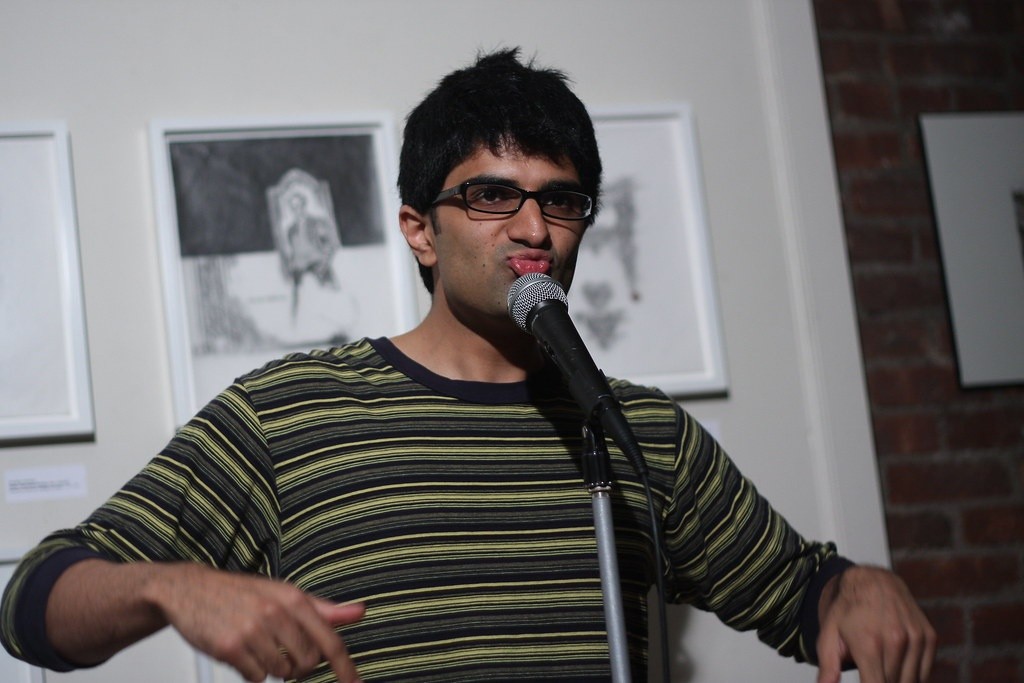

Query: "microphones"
<box><xmin>506</xmin><ymin>272</ymin><xmax>647</xmax><ymax>477</ymax></box>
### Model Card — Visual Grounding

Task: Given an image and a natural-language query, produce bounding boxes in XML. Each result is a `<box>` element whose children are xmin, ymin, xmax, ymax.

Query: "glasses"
<box><xmin>422</xmin><ymin>179</ymin><xmax>593</xmax><ymax>220</ymax></box>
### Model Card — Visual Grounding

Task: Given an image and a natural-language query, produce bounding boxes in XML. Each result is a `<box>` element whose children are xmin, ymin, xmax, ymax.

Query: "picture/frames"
<box><xmin>1</xmin><ymin>121</ymin><xmax>97</xmax><ymax>444</ymax></box>
<box><xmin>566</xmin><ymin>101</ymin><xmax>727</xmax><ymax>398</ymax></box>
<box><xmin>147</xmin><ymin>111</ymin><xmax>422</xmax><ymax>438</ymax></box>
<box><xmin>903</xmin><ymin>100</ymin><xmax>1024</xmax><ymax>396</ymax></box>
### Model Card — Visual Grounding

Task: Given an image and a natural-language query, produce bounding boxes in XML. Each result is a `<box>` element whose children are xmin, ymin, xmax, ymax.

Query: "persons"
<box><xmin>0</xmin><ymin>49</ymin><xmax>938</xmax><ymax>683</ymax></box>
